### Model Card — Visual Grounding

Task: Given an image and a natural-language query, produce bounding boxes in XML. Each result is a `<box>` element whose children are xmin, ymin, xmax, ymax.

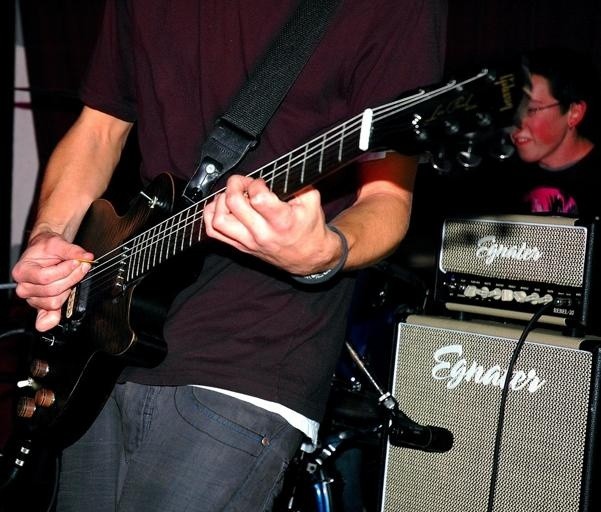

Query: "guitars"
<box><xmin>0</xmin><ymin>56</ymin><xmax>534</xmax><ymax>512</ymax></box>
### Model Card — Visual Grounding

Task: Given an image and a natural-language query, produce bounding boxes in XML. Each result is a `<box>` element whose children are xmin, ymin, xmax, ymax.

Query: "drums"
<box><xmin>269</xmin><ymin>431</ymin><xmax>380</xmax><ymax>512</ymax></box>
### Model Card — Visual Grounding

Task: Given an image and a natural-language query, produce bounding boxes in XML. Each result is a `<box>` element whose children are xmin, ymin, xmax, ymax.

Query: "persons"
<box><xmin>10</xmin><ymin>0</ymin><xmax>445</xmax><ymax>512</ymax></box>
<box><xmin>434</xmin><ymin>48</ymin><xmax>599</xmax><ymax>219</ymax></box>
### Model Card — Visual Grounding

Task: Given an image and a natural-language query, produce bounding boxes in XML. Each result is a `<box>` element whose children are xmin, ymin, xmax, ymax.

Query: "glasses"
<box><xmin>527</xmin><ymin>102</ymin><xmax>560</xmax><ymax>118</ymax></box>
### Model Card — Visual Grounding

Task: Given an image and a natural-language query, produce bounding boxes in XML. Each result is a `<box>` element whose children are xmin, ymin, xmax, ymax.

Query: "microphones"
<box><xmin>374</xmin><ymin>415</ymin><xmax>453</xmax><ymax>453</ymax></box>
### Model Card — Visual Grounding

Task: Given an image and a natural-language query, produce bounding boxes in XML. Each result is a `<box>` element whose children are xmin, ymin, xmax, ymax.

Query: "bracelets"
<box><xmin>288</xmin><ymin>225</ymin><xmax>348</xmax><ymax>285</ymax></box>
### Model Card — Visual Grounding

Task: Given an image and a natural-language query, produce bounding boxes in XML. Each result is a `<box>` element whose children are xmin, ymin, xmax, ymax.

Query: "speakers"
<box><xmin>377</xmin><ymin>213</ymin><xmax>601</xmax><ymax>512</ymax></box>
